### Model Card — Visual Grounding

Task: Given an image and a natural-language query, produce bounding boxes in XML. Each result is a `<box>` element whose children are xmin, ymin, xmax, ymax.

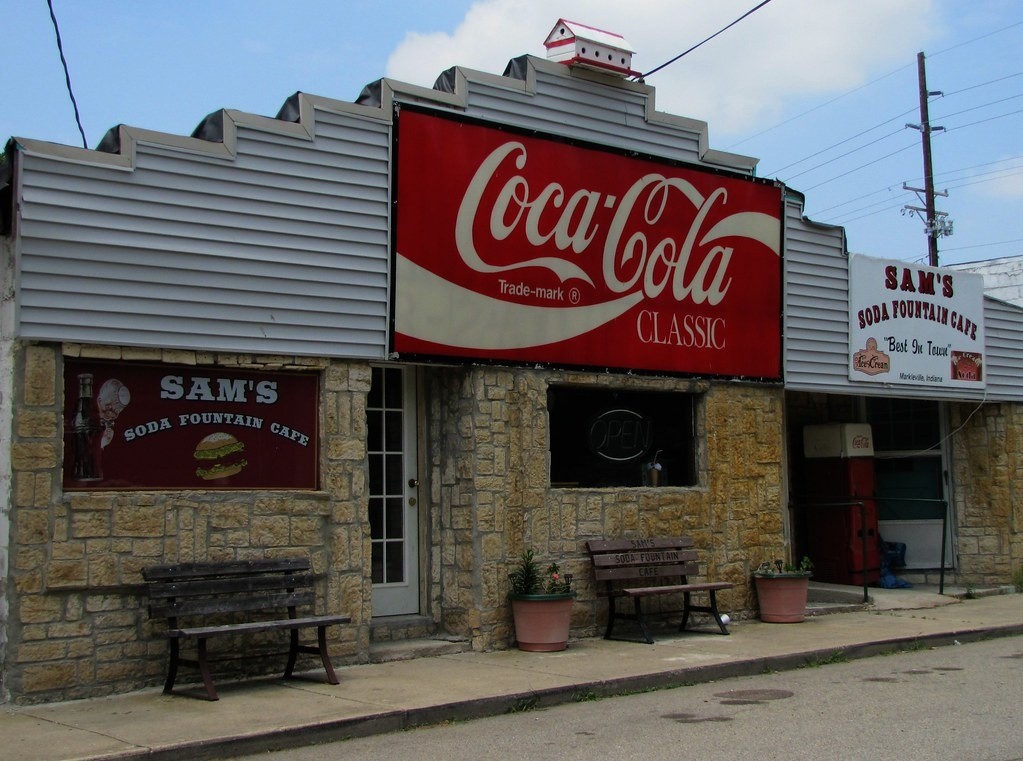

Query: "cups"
<box><xmin>718</xmin><ymin>614</ymin><xmax>731</xmax><ymax>624</ymax></box>
<box><xmin>649</xmin><ymin>468</ymin><xmax>658</xmax><ymax>486</ymax></box>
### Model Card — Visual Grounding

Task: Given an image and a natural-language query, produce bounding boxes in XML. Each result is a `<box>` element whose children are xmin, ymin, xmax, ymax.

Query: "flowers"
<box><xmin>507</xmin><ymin>547</ymin><xmax>571</xmax><ymax>596</ymax></box>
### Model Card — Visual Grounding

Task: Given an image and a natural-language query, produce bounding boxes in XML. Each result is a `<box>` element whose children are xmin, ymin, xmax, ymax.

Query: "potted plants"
<box><xmin>751</xmin><ymin>557</ymin><xmax>815</xmax><ymax>623</ymax></box>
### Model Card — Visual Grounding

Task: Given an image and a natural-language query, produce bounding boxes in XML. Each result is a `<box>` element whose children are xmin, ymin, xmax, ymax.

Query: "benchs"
<box><xmin>140</xmin><ymin>556</ymin><xmax>351</xmax><ymax>701</ymax></box>
<box><xmin>584</xmin><ymin>540</ymin><xmax>735</xmax><ymax>644</ymax></box>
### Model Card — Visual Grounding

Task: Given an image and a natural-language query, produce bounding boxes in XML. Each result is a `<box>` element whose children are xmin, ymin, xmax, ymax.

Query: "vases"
<box><xmin>506</xmin><ymin>592</ymin><xmax>575</xmax><ymax>651</ymax></box>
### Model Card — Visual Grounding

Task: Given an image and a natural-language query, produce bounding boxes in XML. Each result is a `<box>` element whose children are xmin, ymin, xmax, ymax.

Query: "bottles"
<box><xmin>71</xmin><ymin>374</ymin><xmax>101</xmax><ymax>481</ymax></box>
